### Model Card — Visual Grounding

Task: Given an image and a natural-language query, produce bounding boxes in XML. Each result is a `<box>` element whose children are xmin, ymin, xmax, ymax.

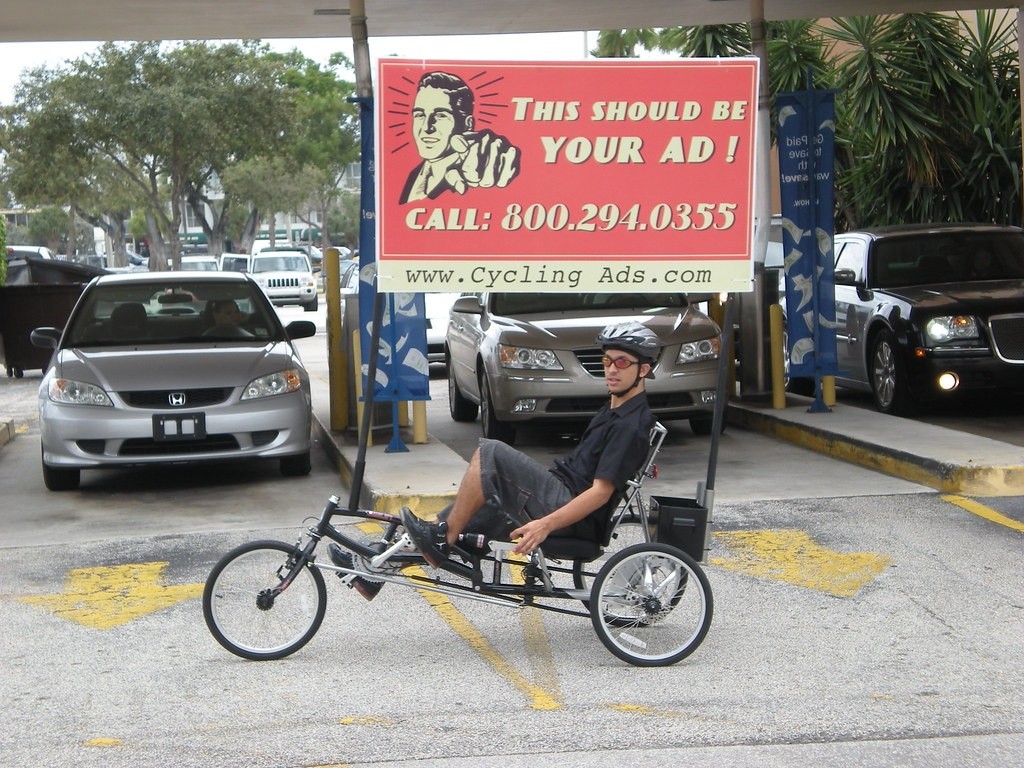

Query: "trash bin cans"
<box><xmin>740</xmin><ymin>270</ymin><xmax>780</xmax><ymax>401</ymax></box>
<box><xmin>342</xmin><ymin>287</ymin><xmax>394</xmax><ymax>432</ymax></box>
<box><xmin>0</xmin><ymin>256</ymin><xmax>117</xmax><ymax>379</ymax></box>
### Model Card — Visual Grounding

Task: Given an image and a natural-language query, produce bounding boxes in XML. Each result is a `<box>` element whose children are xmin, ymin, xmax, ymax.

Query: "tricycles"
<box><xmin>203</xmin><ymin>423</ymin><xmax>713</xmax><ymax>668</ymax></box>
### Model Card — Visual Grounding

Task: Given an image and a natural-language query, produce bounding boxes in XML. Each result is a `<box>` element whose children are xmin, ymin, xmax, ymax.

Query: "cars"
<box><xmin>0</xmin><ymin>245</ymin><xmax>149</xmax><ymax>378</ymax></box>
<box><xmin>326</xmin><ymin>262</ymin><xmax>461</xmax><ymax>363</ymax></box>
<box><xmin>777</xmin><ymin>222</ymin><xmax>1023</xmax><ymax>417</ymax></box>
<box><xmin>171</xmin><ymin>256</ymin><xmax>219</xmax><ymax>272</ymax></box>
<box><xmin>318</xmin><ymin>246</ymin><xmax>353</xmax><ymax>260</ymax></box>
<box><xmin>319</xmin><ymin>259</ymin><xmax>353</xmax><ymax>290</ymax></box>
<box><xmin>30</xmin><ymin>271</ymin><xmax>316</xmax><ymax>491</ymax></box>
<box><xmin>694</xmin><ymin>214</ymin><xmax>785</xmax><ymax>380</ymax></box>
<box><xmin>164</xmin><ymin>240</ymin><xmax>186</xmax><ymax>267</ymax></box>
<box><xmin>445</xmin><ymin>293</ymin><xmax>737</xmax><ymax>445</ymax></box>
<box><xmin>253</xmin><ymin>244</ymin><xmax>323</xmax><ymax>272</ymax></box>
<box><xmin>221</xmin><ymin>250</ymin><xmax>318</xmax><ymax>312</ymax></box>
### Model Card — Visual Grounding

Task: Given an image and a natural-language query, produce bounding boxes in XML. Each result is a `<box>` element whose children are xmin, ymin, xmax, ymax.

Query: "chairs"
<box><xmin>108</xmin><ymin>303</ymin><xmax>147</xmax><ymax>339</ymax></box>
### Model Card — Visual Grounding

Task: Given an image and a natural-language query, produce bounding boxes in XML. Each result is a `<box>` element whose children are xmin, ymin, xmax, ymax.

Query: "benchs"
<box><xmin>883</xmin><ymin>255</ymin><xmax>937</xmax><ymax>284</ymax></box>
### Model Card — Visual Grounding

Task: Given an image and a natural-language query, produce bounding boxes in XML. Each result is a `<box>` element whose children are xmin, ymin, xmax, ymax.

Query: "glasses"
<box><xmin>601</xmin><ymin>355</ymin><xmax>638</xmax><ymax>369</ymax></box>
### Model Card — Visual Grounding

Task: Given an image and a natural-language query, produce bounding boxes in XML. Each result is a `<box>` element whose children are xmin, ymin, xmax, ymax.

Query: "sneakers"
<box><xmin>399</xmin><ymin>507</ymin><xmax>449</xmax><ymax>570</ymax></box>
<box><xmin>326</xmin><ymin>543</ymin><xmax>386</xmax><ymax>601</ymax></box>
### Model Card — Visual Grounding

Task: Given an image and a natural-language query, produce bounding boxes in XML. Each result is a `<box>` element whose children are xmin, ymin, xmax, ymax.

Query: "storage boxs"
<box><xmin>648</xmin><ymin>496</ymin><xmax>709</xmax><ymax>563</ymax></box>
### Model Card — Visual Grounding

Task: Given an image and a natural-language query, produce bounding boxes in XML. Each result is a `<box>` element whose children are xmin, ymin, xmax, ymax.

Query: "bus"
<box><xmin>165</xmin><ymin>223</ymin><xmax>322</xmax><ymax>247</ymax></box>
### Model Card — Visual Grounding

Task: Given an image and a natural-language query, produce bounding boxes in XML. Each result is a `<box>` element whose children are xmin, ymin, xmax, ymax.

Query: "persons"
<box><xmin>326</xmin><ymin>322</ymin><xmax>660</xmax><ymax>602</ymax></box>
<box><xmin>197</xmin><ymin>300</ymin><xmax>257</xmax><ymax>338</ymax></box>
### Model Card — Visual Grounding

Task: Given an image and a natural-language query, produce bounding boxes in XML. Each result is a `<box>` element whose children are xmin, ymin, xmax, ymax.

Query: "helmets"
<box><xmin>596</xmin><ymin>320</ymin><xmax>661</xmax><ymax>363</ymax></box>
